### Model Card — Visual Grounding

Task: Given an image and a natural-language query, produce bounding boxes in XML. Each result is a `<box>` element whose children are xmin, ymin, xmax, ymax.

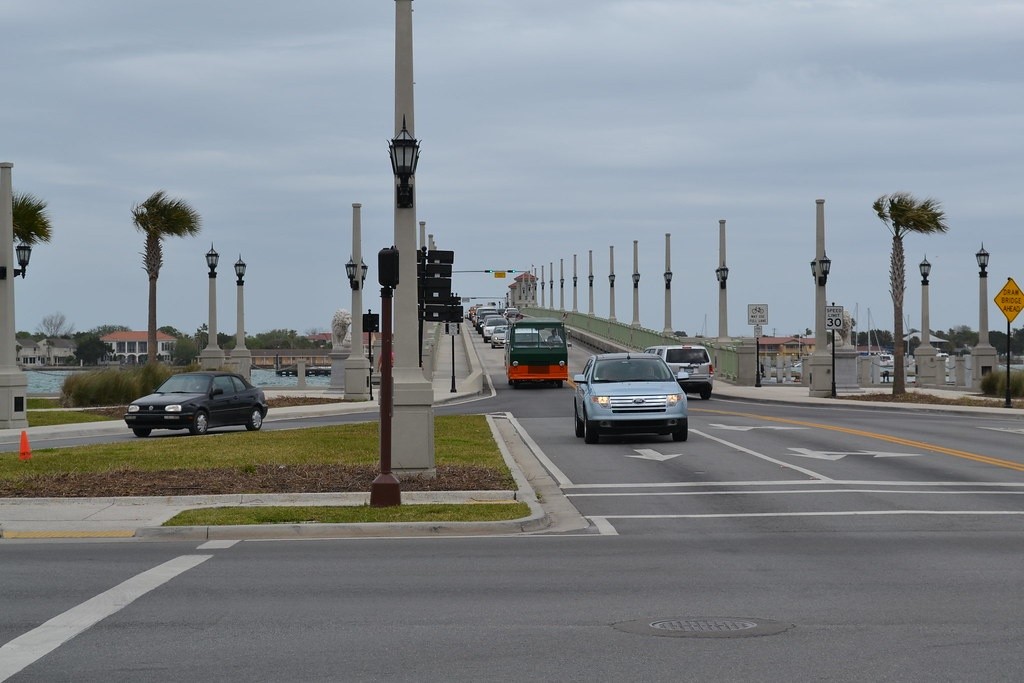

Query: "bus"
<box><xmin>502</xmin><ymin>317</ymin><xmax>568</xmax><ymax>388</ymax></box>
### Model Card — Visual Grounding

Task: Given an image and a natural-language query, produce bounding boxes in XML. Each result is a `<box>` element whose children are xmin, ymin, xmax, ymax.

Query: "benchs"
<box><xmin>771</xmin><ymin>372</ymin><xmax>802</xmax><ymax>382</ymax></box>
<box><xmin>515</xmin><ymin>333</ymin><xmax>543</xmax><ymax>342</ymax></box>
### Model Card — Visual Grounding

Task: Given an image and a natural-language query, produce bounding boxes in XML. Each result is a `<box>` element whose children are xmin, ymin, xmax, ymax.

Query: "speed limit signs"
<box><xmin>826</xmin><ymin>306</ymin><xmax>842</xmax><ymax>329</ymax></box>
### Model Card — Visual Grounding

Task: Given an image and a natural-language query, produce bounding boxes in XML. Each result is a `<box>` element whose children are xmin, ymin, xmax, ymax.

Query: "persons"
<box><xmin>547</xmin><ymin>330</ymin><xmax>563</xmax><ymax>343</ymax></box>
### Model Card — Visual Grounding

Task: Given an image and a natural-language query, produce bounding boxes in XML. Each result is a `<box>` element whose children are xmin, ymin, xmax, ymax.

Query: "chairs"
<box><xmin>638</xmin><ymin>363</ymin><xmax>659</xmax><ymax>379</ymax></box>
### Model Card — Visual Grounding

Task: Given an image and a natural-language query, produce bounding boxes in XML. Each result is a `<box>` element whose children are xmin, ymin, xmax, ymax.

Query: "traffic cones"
<box><xmin>19</xmin><ymin>430</ymin><xmax>31</xmax><ymax>461</ymax></box>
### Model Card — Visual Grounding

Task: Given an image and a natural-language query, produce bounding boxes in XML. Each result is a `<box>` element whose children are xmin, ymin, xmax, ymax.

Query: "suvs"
<box><xmin>642</xmin><ymin>345</ymin><xmax>714</xmax><ymax>399</ymax></box>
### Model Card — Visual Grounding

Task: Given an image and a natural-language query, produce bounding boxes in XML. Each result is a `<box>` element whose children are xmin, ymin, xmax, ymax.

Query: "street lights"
<box><xmin>0</xmin><ymin>238</ymin><xmax>32</xmax><ymax>429</ymax></box>
<box><xmin>528</xmin><ymin>249</ymin><xmax>595</xmax><ymax>316</ymax></box>
<box><xmin>808</xmin><ymin>251</ymin><xmax>832</xmax><ymax>396</ymax></box>
<box><xmin>914</xmin><ymin>254</ymin><xmax>936</xmax><ymax>385</ymax></box>
<box><xmin>716</xmin><ymin>262</ymin><xmax>729</xmax><ymax>342</ymax></box>
<box><xmin>230</xmin><ymin>253</ymin><xmax>252</xmax><ymax>377</ymax></box>
<box><xmin>632</xmin><ymin>270</ymin><xmax>641</xmax><ymax>327</ymax></box>
<box><xmin>970</xmin><ymin>242</ymin><xmax>998</xmax><ymax>390</ymax></box>
<box><xmin>607</xmin><ymin>271</ymin><xmax>617</xmax><ymax>321</ymax></box>
<box><xmin>663</xmin><ymin>268</ymin><xmax>673</xmax><ymax>336</ymax></box>
<box><xmin>343</xmin><ymin>254</ymin><xmax>371</xmax><ymax>402</ymax></box>
<box><xmin>388</xmin><ymin>115</ymin><xmax>439</xmax><ymax>481</ymax></box>
<box><xmin>193</xmin><ymin>244</ymin><xmax>230</xmax><ymax>369</ymax></box>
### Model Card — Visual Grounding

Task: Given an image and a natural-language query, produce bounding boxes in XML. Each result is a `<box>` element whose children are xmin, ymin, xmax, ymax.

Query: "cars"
<box><xmin>574</xmin><ymin>352</ymin><xmax>690</xmax><ymax>443</ymax></box>
<box><xmin>124</xmin><ymin>371</ymin><xmax>269</xmax><ymax>438</ymax></box>
<box><xmin>466</xmin><ymin>306</ymin><xmax>543</xmax><ymax>348</ymax></box>
